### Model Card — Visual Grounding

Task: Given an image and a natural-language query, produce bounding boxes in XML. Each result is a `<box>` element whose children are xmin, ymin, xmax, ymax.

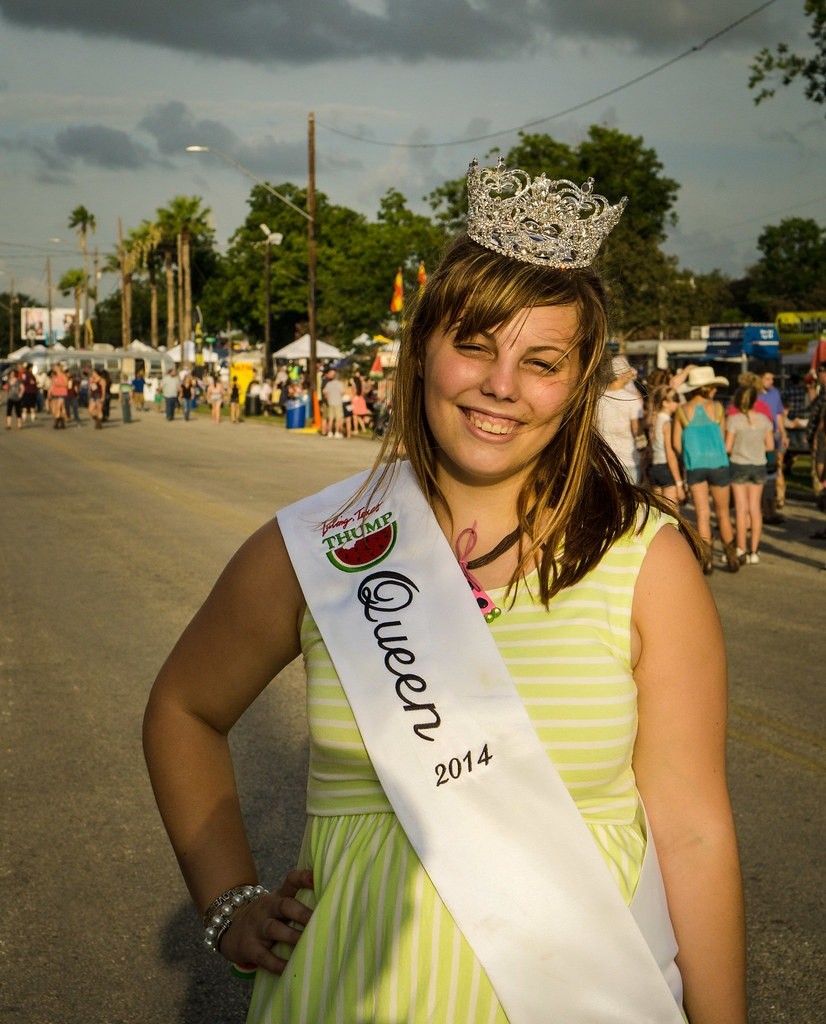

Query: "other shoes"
<box><xmin>746</xmin><ymin>551</ymin><xmax>761</xmax><ymax>564</ymax></box>
<box><xmin>327</xmin><ymin>430</ymin><xmax>344</xmax><ymax>439</ymax></box>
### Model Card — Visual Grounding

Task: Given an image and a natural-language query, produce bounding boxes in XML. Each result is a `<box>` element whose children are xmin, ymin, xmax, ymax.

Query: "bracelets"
<box><xmin>201</xmin><ymin>882</ymin><xmax>275</xmax><ymax>956</ymax></box>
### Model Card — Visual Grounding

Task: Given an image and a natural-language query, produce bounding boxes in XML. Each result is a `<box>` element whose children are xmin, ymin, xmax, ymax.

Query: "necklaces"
<box><xmin>420</xmin><ymin>440</ymin><xmax>570</xmax><ymax>625</ymax></box>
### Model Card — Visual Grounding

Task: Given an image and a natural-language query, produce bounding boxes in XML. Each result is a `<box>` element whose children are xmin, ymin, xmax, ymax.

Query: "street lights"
<box><xmin>185</xmin><ymin>144</ymin><xmax>318</xmax><ymax>427</ymax></box>
<box><xmin>49</xmin><ymin>237</ymin><xmax>98</xmax><ymax>346</ymax></box>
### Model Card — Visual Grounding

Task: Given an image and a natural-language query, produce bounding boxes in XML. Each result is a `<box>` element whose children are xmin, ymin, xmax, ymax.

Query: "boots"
<box><xmin>92</xmin><ymin>415</ymin><xmax>103</xmax><ymax>429</ymax></box>
<box><xmin>54</xmin><ymin>417</ymin><xmax>66</xmax><ymax>430</ymax></box>
<box><xmin>721</xmin><ymin>537</ymin><xmax>739</xmax><ymax>572</ymax></box>
<box><xmin>702</xmin><ymin>541</ymin><xmax>714</xmax><ymax>575</ymax></box>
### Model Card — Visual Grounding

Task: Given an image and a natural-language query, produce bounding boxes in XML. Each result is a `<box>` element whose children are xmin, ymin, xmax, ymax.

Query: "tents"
<box><xmin>271</xmin><ymin>333</ymin><xmax>347</xmax><ymax>360</ymax></box>
<box><xmin>0</xmin><ymin>338</ymin><xmax>267</xmax><ymax>363</ymax></box>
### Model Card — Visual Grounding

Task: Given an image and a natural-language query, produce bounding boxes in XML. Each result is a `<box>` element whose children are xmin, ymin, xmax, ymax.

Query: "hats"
<box><xmin>677</xmin><ymin>366</ymin><xmax>730</xmax><ymax>394</ymax></box>
<box><xmin>612</xmin><ymin>358</ymin><xmax>632</xmax><ymax>377</ymax></box>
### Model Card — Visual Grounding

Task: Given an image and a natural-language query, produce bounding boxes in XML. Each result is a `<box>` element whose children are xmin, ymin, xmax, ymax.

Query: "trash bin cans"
<box><xmin>286</xmin><ymin>396</ymin><xmax>305</xmax><ymax>429</ymax></box>
<box><xmin>245</xmin><ymin>395</ymin><xmax>262</xmax><ymax>416</ymax></box>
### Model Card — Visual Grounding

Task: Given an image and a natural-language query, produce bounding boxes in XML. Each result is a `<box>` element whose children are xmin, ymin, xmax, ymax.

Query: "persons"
<box><xmin>591</xmin><ymin>354</ymin><xmax>826</xmax><ymax>577</ymax></box>
<box><xmin>140</xmin><ymin>155</ymin><xmax>747</xmax><ymax>1024</ymax></box>
<box><xmin>0</xmin><ymin>361</ymin><xmax>398</xmax><ymax>439</ymax></box>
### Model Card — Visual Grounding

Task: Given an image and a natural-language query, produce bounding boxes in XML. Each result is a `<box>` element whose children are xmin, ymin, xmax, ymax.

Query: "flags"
<box><xmin>390</xmin><ymin>267</ymin><xmax>405</xmax><ymax>314</ymax></box>
<box><xmin>416</xmin><ymin>260</ymin><xmax>429</xmax><ymax>302</ymax></box>
